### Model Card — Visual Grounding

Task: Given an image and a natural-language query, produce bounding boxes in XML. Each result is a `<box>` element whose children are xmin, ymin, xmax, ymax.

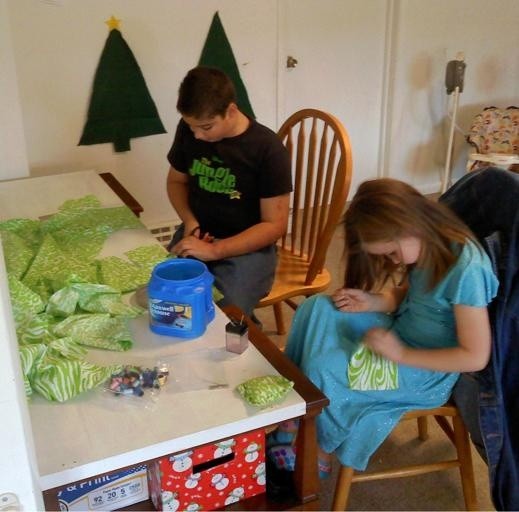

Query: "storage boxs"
<box><xmin>57</xmin><ymin>431</ymin><xmax>267</xmax><ymax>512</ymax></box>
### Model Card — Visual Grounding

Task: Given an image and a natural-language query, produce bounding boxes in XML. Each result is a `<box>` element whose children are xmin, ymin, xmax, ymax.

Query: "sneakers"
<box><xmin>268</xmin><ymin>444</ymin><xmax>333</xmax><ymax>481</ymax></box>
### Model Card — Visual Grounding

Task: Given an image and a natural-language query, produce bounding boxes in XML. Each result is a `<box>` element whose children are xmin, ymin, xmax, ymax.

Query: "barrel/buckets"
<box><xmin>146</xmin><ymin>259</ymin><xmax>216</xmax><ymax>337</ymax></box>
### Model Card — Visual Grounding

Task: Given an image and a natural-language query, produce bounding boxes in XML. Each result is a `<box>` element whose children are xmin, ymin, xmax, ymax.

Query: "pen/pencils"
<box><xmin>230</xmin><ymin>313</ymin><xmax>244</xmax><ymax>326</ymax></box>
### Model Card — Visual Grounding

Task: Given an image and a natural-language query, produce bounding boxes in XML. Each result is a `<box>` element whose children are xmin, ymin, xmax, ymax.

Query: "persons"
<box><xmin>166</xmin><ymin>66</ymin><xmax>292</xmax><ymax>324</ymax></box>
<box><xmin>265</xmin><ymin>179</ymin><xmax>501</xmax><ymax>479</ymax></box>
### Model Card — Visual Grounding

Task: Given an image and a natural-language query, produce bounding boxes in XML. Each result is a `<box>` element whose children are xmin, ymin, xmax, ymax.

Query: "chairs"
<box><xmin>327</xmin><ymin>167</ymin><xmax>518</xmax><ymax>511</ymax></box>
<box><xmin>254</xmin><ymin>104</ymin><xmax>354</xmax><ymax>334</ymax></box>
